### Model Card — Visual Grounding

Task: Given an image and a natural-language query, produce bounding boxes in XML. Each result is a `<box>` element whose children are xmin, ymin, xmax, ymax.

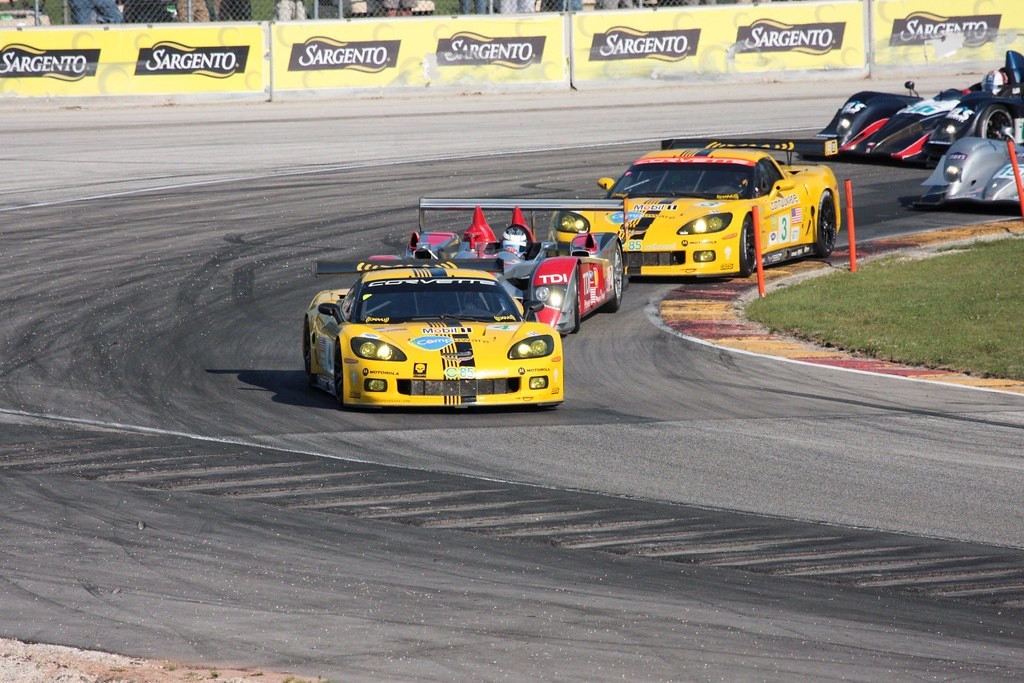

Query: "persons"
<box><xmin>218</xmin><ymin>0</ymin><xmax>252</xmax><ymax>20</ymax></box>
<box><xmin>121</xmin><ymin>0</ymin><xmax>174</xmax><ymax>23</ymax></box>
<box><xmin>501</xmin><ymin>228</ymin><xmax>527</xmax><ymax>259</ymax></box>
<box><xmin>176</xmin><ymin>0</ymin><xmax>210</xmax><ymax>22</ymax></box>
<box><xmin>498</xmin><ymin>0</ymin><xmax>772</xmax><ymax>13</ymax></box>
<box><xmin>982</xmin><ymin>71</ymin><xmax>1008</xmax><ymax>96</ymax></box>
<box><xmin>383</xmin><ymin>0</ymin><xmax>418</xmax><ymax>16</ymax></box>
<box><xmin>67</xmin><ymin>0</ymin><xmax>123</xmax><ymax>24</ymax></box>
<box><xmin>461</xmin><ymin>0</ymin><xmax>486</xmax><ymax>15</ymax></box>
<box><xmin>276</xmin><ymin>0</ymin><xmax>306</xmax><ymax>21</ymax></box>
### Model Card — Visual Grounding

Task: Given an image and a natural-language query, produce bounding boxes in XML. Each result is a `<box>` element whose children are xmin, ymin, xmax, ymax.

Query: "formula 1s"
<box><xmin>366</xmin><ymin>195</ymin><xmax>626</xmax><ymax>336</ymax></box>
<box><xmin>914</xmin><ymin>118</ymin><xmax>1024</xmax><ymax>210</ymax></box>
<box><xmin>814</xmin><ymin>50</ymin><xmax>1024</xmax><ymax>169</ymax></box>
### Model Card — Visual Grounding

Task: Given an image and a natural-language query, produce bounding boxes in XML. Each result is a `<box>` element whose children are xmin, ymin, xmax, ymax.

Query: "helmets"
<box><xmin>981</xmin><ymin>71</ymin><xmax>1003</xmax><ymax>94</ymax></box>
<box><xmin>500</xmin><ymin>225</ymin><xmax>532</xmax><ymax>256</ymax></box>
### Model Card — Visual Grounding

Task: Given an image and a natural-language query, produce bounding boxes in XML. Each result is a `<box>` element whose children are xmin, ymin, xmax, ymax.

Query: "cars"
<box><xmin>597</xmin><ymin>134</ymin><xmax>841</xmax><ymax>281</ymax></box>
<box><xmin>303</xmin><ymin>259</ymin><xmax>565</xmax><ymax>412</ymax></box>
